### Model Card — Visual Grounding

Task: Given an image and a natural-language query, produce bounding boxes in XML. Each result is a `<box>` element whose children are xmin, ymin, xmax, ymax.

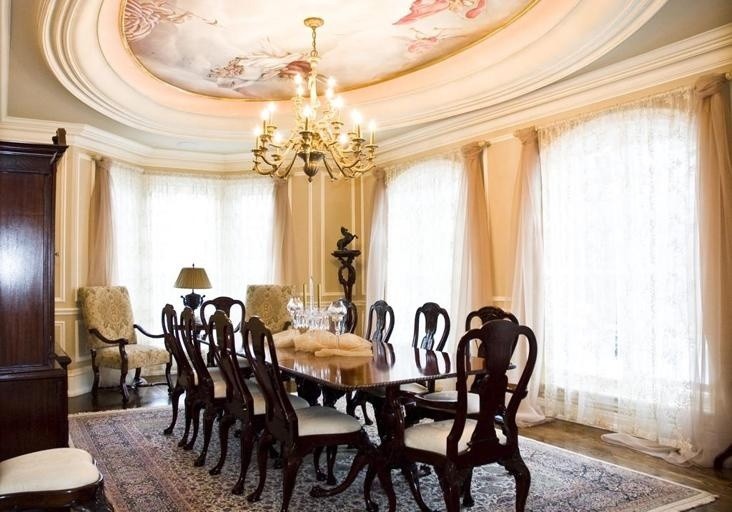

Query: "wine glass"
<box><xmin>326</xmin><ymin>299</ymin><xmax>349</xmax><ymax>357</ymax></box>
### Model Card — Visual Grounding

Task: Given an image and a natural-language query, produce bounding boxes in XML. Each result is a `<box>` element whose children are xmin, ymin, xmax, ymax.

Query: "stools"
<box><xmin>0</xmin><ymin>446</ymin><xmax>117</xmax><ymax>512</ymax></box>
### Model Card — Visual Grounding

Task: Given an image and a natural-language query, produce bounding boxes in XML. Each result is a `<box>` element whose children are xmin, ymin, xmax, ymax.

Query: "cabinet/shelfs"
<box><xmin>0</xmin><ymin>126</ymin><xmax>71</xmax><ymax>460</ymax></box>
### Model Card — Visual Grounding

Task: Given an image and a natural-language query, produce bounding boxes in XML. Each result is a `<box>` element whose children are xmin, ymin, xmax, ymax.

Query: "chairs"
<box><xmin>360</xmin><ymin>299</ymin><xmax>396</xmax><ymax>345</ymax></box>
<box><xmin>330</xmin><ymin>297</ymin><xmax>359</xmax><ymax>334</ymax></box>
<box><xmin>361</xmin><ymin>300</ymin><xmax>455</xmax><ymax>427</ymax></box>
<box><xmin>414</xmin><ymin>305</ymin><xmax>520</xmax><ymax>421</ymax></box>
<box><xmin>241</xmin><ymin>315</ymin><xmax>376</xmax><ymax>511</ymax></box>
<box><xmin>158</xmin><ymin>296</ymin><xmax>246</xmax><ymax>468</ymax></box>
<box><xmin>403</xmin><ymin>318</ymin><xmax>538</xmax><ymax>512</ymax></box>
<box><xmin>79</xmin><ymin>286</ymin><xmax>173</xmax><ymax>403</ymax></box>
<box><xmin>208</xmin><ymin>309</ymin><xmax>310</xmax><ymax>497</ymax></box>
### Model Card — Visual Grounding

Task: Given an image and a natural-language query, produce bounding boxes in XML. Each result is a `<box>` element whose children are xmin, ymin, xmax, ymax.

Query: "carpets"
<box><xmin>67</xmin><ymin>390</ymin><xmax>721</xmax><ymax>511</ymax></box>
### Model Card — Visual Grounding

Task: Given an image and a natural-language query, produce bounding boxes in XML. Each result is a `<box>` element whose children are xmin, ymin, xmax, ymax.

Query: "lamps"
<box><xmin>250</xmin><ymin>17</ymin><xmax>379</xmax><ymax>183</ymax></box>
<box><xmin>173</xmin><ymin>263</ymin><xmax>213</xmax><ymax>322</ymax></box>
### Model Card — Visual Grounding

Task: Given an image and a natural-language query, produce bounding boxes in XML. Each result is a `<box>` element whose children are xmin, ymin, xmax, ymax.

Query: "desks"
<box><xmin>186</xmin><ymin>328</ymin><xmax>517</xmax><ymax>511</ymax></box>
